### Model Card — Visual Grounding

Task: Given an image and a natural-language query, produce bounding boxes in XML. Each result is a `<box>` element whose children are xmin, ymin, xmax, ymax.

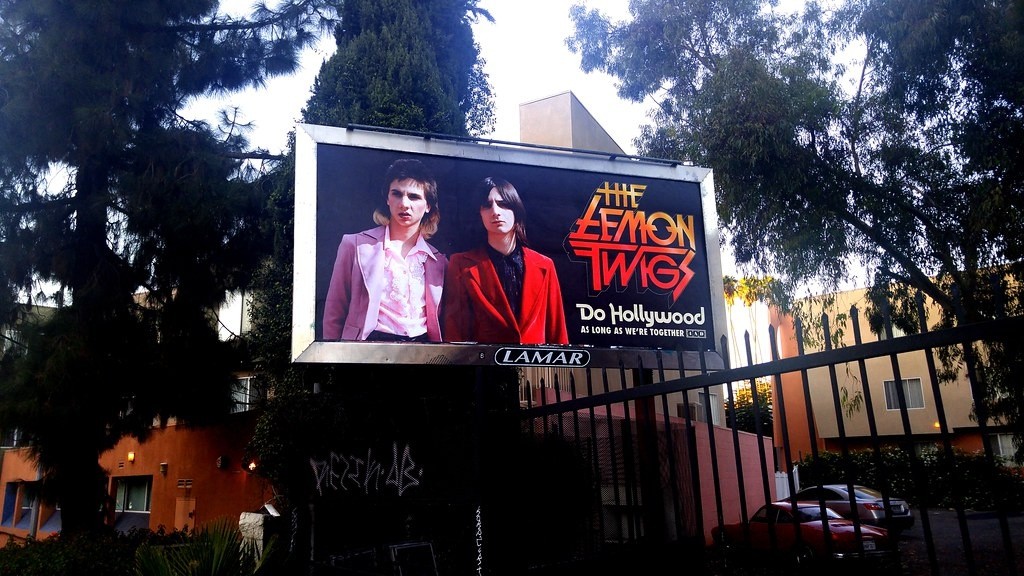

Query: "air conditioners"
<box><xmin>677</xmin><ymin>401</ymin><xmax>704</xmax><ymax>423</ymax></box>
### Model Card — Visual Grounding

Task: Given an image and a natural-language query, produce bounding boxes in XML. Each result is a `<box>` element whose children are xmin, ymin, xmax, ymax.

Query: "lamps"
<box><xmin>128</xmin><ymin>451</ymin><xmax>135</xmax><ymax>462</ymax></box>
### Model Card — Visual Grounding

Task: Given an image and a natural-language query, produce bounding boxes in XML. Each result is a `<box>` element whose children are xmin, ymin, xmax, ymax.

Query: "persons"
<box><xmin>323</xmin><ymin>160</ymin><xmax>449</xmax><ymax>344</ymax></box>
<box><xmin>444</xmin><ymin>176</ymin><xmax>568</xmax><ymax>344</ymax></box>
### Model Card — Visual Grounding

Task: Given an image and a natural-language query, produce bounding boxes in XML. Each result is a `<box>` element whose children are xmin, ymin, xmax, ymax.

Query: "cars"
<box><xmin>709</xmin><ymin>500</ymin><xmax>893</xmax><ymax>571</ymax></box>
<box><xmin>773</xmin><ymin>482</ymin><xmax>915</xmax><ymax>542</ymax></box>
